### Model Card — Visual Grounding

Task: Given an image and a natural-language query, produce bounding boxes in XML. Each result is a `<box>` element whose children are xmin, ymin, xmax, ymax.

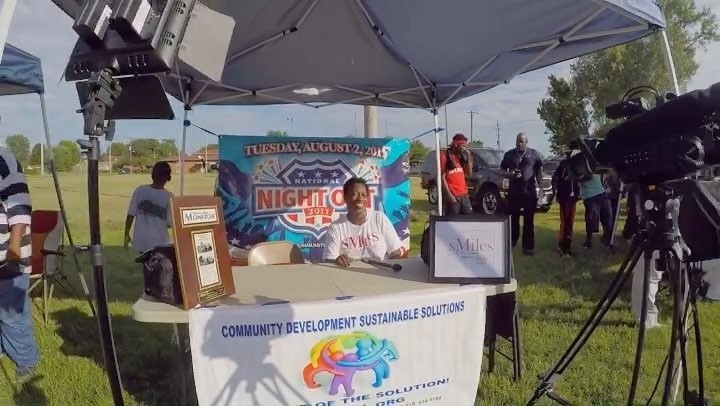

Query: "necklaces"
<box><xmin>514</xmin><ymin>147</ymin><xmax>527</xmax><ymax>163</ymax></box>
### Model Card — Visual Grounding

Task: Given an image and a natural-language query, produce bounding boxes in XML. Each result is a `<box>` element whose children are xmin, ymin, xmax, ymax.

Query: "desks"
<box><xmin>129</xmin><ymin>256</ymin><xmax>520</xmax><ymax>406</ymax></box>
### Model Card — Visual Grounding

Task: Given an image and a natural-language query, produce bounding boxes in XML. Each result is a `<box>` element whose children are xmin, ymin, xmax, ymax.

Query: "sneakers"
<box><xmin>17</xmin><ymin>366</ymin><xmax>32</xmax><ymax>378</ymax></box>
<box><xmin>522</xmin><ymin>247</ymin><xmax>534</xmax><ymax>256</ymax></box>
<box><xmin>558</xmin><ymin>243</ymin><xmax>572</xmax><ymax>258</ymax></box>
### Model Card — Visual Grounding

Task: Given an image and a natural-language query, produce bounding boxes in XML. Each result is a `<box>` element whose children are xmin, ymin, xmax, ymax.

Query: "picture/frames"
<box><xmin>428</xmin><ymin>214</ymin><xmax>514</xmax><ymax>283</ymax></box>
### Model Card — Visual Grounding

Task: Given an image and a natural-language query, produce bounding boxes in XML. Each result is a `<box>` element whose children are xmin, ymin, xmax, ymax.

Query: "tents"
<box><xmin>0</xmin><ymin>40</ymin><xmax>100</xmax><ymax>335</ymax></box>
<box><xmin>50</xmin><ymin>1</ymin><xmax>680</xmax><ymax>405</ymax></box>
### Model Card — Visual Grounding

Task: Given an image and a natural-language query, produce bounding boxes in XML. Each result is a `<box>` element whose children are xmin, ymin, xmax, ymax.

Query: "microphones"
<box><xmin>366</xmin><ymin>259</ymin><xmax>402</xmax><ymax>273</ymax></box>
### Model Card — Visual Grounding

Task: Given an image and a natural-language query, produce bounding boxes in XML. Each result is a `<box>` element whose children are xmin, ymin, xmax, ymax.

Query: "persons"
<box><xmin>0</xmin><ymin>145</ymin><xmax>43</xmax><ymax>383</ymax></box>
<box><xmin>582</xmin><ymin>162</ymin><xmax>617</xmax><ymax>254</ymax></box>
<box><xmin>440</xmin><ymin>133</ymin><xmax>476</xmax><ymax>216</ymax></box>
<box><xmin>123</xmin><ymin>160</ymin><xmax>177</xmax><ymax>261</ymax></box>
<box><xmin>546</xmin><ymin>143</ymin><xmax>585</xmax><ymax>259</ymax></box>
<box><xmin>320</xmin><ymin>176</ymin><xmax>404</xmax><ymax>268</ymax></box>
<box><xmin>620</xmin><ymin>177</ymin><xmax>663</xmax><ymax>333</ymax></box>
<box><xmin>498</xmin><ymin>132</ymin><xmax>546</xmax><ymax>256</ymax></box>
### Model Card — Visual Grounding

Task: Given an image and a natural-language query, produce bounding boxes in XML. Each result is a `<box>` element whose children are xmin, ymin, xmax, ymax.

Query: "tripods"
<box><xmin>60</xmin><ymin>68</ymin><xmax>128</xmax><ymax>405</ymax></box>
<box><xmin>520</xmin><ymin>180</ymin><xmax>719</xmax><ymax>406</ymax></box>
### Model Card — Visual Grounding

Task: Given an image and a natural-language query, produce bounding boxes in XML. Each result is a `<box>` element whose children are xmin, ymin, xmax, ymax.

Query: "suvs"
<box><xmin>420</xmin><ymin>147</ymin><xmax>554</xmax><ymax>218</ymax></box>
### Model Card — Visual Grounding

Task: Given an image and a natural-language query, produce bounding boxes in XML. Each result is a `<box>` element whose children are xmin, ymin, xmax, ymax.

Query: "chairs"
<box><xmin>27</xmin><ymin>209</ymin><xmax>64</xmax><ymax>323</ymax></box>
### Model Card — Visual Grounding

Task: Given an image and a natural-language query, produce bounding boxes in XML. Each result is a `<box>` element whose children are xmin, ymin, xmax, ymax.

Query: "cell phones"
<box><xmin>515</xmin><ymin>170</ymin><xmax>521</xmax><ymax>174</ymax></box>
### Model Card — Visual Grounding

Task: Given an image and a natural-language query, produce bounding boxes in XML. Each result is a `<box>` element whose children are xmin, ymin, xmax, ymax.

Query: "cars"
<box><xmin>541</xmin><ymin>159</ymin><xmax>561</xmax><ymax>175</ymax></box>
